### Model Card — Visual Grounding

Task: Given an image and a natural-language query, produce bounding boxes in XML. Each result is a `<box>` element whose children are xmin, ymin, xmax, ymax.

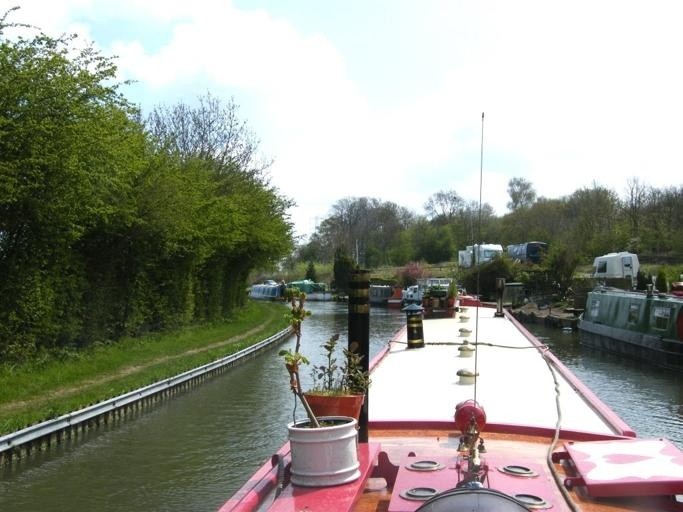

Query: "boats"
<box><xmin>370</xmin><ymin>277</ymin><xmax>467</xmax><ymax>310</ymax></box>
<box><xmin>251</xmin><ymin>280</ymin><xmax>332</xmax><ymax>301</ymax></box>
<box><xmin>577</xmin><ymin>284</ymin><xmax>683</xmax><ymax>372</ymax></box>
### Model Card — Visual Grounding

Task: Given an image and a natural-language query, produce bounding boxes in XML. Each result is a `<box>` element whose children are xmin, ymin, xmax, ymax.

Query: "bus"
<box><xmin>458</xmin><ymin>241</ymin><xmax>550</xmax><ymax>271</ymax></box>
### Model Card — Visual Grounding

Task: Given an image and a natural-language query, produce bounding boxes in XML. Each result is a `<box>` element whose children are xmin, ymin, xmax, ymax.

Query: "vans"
<box><xmin>592</xmin><ymin>251</ymin><xmax>640</xmax><ymax>280</ymax></box>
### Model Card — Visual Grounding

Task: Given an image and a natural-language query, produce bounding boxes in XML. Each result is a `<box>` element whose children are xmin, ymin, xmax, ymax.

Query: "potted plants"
<box><xmin>279</xmin><ymin>283</ymin><xmax>362</xmax><ymax>488</ymax></box>
<box><xmin>303</xmin><ymin>333</ymin><xmax>371</xmax><ymax>421</ymax></box>
<box><xmin>421</xmin><ymin>281</ymin><xmax>457</xmax><ymax>308</ymax></box>
